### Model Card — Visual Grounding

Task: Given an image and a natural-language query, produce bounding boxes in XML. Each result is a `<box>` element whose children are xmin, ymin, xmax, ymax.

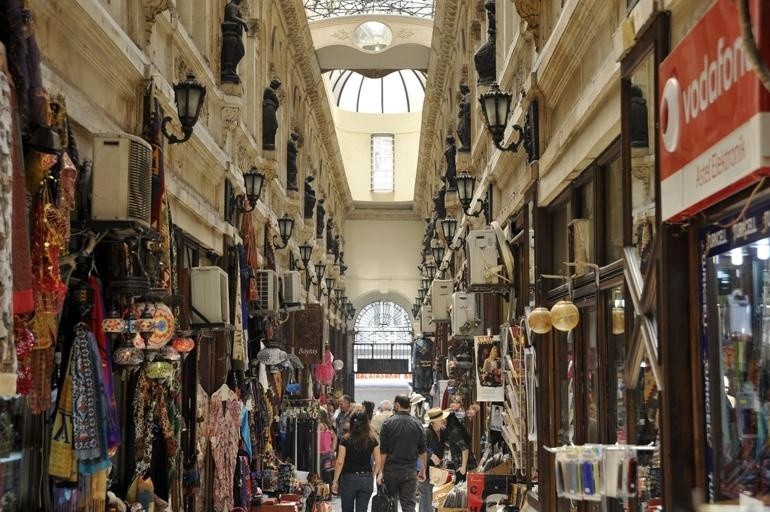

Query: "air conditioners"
<box><xmin>465</xmin><ymin>229</ymin><xmax>499</xmax><ymax>287</ymax></box>
<box><xmin>419</xmin><ymin>279</ymin><xmax>478</xmax><ymax>340</ymax></box>
<box><xmin>191</xmin><ymin>266</ymin><xmax>230</xmax><ymax>331</ymax></box>
<box><xmin>91</xmin><ymin>132</ymin><xmax>153</xmax><ymax>229</ymax></box>
<box><xmin>251</xmin><ymin>269</ymin><xmax>279</xmax><ymax>318</ymax></box>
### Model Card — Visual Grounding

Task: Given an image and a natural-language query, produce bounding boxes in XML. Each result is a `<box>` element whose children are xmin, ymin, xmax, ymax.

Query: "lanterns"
<box><xmin>527</xmin><ymin>307</ymin><xmax>551</xmax><ymax>335</ymax></box>
<box><xmin>550</xmin><ymin>300</ymin><xmax>579</xmax><ymax>332</ymax></box>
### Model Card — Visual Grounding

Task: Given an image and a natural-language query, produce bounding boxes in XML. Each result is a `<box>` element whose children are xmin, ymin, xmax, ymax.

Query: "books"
<box><xmin>500</xmin><ymin>325</ymin><xmax>524</xmax><ymax>469</ymax></box>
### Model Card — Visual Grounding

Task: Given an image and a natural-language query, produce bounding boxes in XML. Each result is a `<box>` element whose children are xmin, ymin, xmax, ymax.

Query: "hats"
<box><xmin>411</xmin><ymin>393</ymin><xmax>427</xmax><ymax>405</ymax></box>
<box><xmin>423</xmin><ymin>407</ymin><xmax>450</xmax><ymax>422</ymax></box>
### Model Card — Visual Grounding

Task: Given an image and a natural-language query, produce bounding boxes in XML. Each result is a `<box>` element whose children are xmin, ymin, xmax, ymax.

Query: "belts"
<box><xmin>347</xmin><ymin>471</ymin><xmax>371</xmax><ymax>474</ymax></box>
<box><xmin>280</xmin><ymin>407</ymin><xmax>317</xmax><ymax>474</ymax></box>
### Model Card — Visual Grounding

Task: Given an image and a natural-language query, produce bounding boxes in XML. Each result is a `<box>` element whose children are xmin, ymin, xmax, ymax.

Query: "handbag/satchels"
<box><xmin>372</xmin><ymin>493</ymin><xmax>394</xmax><ymax>512</ymax></box>
<box><xmin>428</xmin><ymin>465</ymin><xmax>452</xmax><ymax>487</ymax></box>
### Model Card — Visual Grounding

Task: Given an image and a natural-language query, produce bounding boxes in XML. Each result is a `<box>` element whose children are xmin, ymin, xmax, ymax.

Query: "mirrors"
<box><xmin>194</xmin><ymin>336</ymin><xmax>214</xmax><ymax>512</ymax></box>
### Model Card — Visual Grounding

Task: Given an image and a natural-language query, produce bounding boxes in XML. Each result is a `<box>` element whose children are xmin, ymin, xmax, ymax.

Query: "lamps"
<box><xmin>478</xmin><ymin>81</ymin><xmax>540</xmax><ymax>165</ymax></box>
<box><xmin>232</xmin><ymin>166</ymin><xmax>266</xmax><ymax>214</ymax></box>
<box><xmin>154</xmin><ymin>67</ymin><xmax>207</xmax><ymax>145</ymax></box>
<box><xmin>528</xmin><ymin>262</ymin><xmax>600</xmax><ymax>334</ymax></box>
<box><xmin>273</xmin><ymin>212</ymin><xmax>356</xmax><ymax>320</ymax></box>
<box><xmin>422</xmin><ymin>167</ymin><xmax>492</xmax><ymax>292</ymax></box>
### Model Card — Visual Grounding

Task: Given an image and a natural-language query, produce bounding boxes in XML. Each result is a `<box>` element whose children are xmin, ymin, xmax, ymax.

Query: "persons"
<box><xmin>318</xmin><ymin>393</ymin><xmax>477</xmax><ymax>495</ymax></box>
<box><xmin>418</xmin><ymin>83</ymin><xmax>471</xmax><ymax>264</ymax></box>
<box><xmin>376</xmin><ymin>393</ymin><xmax>427</xmax><ymax>511</ymax></box>
<box><xmin>286</xmin><ymin>132</ymin><xmax>348</xmax><ymax>272</ymax></box>
<box><xmin>328</xmin><ymin>406</ymin><xmax>382</xmax><ymax>511</ymax></box>
<box><xmin>220</xmin><ymin>1</ymin><xmax>249</xmax><ymax>82</ymax></box>
<box><xmin>262</xmin><ymin>78</ymin><xmax>282</xmax><ymax>150</ymax></box>
<box><xmin>416</xmin><ymin>408</ymin><xmax>448</xmax><ymax>511</ymax></box>
<box><xmin>492</xmin><ymin>406</ymin><xmax>502</xmax><ymax>427</ymax></box>
<box><xmin>481</xmin><ymin>344</ymin><xmax>500</xmax><ymax>381</ymax></box>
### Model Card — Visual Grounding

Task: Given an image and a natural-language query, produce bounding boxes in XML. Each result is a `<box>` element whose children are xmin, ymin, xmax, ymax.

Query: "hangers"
<box><xmin>220</xmin><ymin>376</ymin><xmax>230</xmax><ymax>402</ymax></box>
